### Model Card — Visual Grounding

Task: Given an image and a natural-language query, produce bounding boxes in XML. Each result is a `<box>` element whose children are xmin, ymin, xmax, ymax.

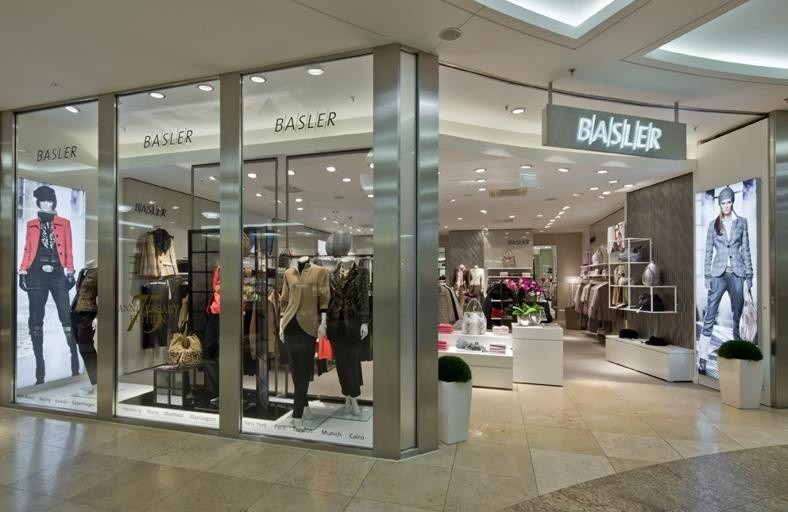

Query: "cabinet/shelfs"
<box><xmin>438</xmin><ymin>326</ymin><xmax>563</xmax><ymax>390</ymax></box>
<box><xmin>609</xmin><ymin>237</ymin><xmax>677</xmax><ymax>314</ymax></box>
<box><xmin>154</xmin><ymin>365</ymin><xmax>208</xmax><ymax>410</ymax></box>
<box><xmin>566</xmin><ymin>307</ymin><xmax>587</xmax><ymax>330</ymax></box>
<box><xmin>486</xmin><ymin>268</ymin><xmax>534</xmax><ymax>326</ymax></box>
<box><xmin>580</xmin><ymin>265</ymin><xmax>608</xmax><ymax>284</ymax></box>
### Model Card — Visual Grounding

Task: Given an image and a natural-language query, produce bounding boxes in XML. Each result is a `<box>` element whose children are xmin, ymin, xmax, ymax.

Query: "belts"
<box><xmin>38</xmin><ymin>256</ymin><xmax>58</xmax><ymax>263</ymax></box>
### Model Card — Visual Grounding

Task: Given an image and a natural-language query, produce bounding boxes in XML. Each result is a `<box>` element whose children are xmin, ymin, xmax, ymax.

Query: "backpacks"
<box><xmin>535</xmin><ymin>290</ymin><xmax>553</xmax><ymax>323</ymax></box>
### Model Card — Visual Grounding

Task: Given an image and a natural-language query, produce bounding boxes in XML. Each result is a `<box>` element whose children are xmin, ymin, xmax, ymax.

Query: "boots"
<box><xmin>62</xmin><ymin>322</ymin><xmax>80</xmax><ymax>378</ymax></box>
<box><xmin>28</xmin><ymin>317</ymin><xmax>45</xmax><ymax>385</ymax></box>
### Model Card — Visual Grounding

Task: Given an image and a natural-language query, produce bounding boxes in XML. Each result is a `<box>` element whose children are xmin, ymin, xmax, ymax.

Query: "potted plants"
<box><xmin>512</xmin><ymin>302</ymin><xmax>547</xmax><ymax>326</ymax></box>
<box><xmin>717</xmin><ymin>339</ymin><xmax>764</xmax><ymax>409</ymax></box>
<box><xmin>438</xmin><ymin>355</ymin><xmax>473</xmax><ymax>445</ymax></box>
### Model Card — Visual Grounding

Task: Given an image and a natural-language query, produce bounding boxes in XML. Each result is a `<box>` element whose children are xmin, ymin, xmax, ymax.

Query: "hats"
<box><xmin>32</xmin><ymin>186</ymin><xmax>56</xmax><ymax>201</ymax></box>
<box><xmin>718</xmin><ymin>187</ymin><xmax>735</xmax><ymax>204</ymax></box>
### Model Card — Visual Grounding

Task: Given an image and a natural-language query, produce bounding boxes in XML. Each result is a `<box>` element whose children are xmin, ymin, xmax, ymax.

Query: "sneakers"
<box><xmin>698</xmin><ymin>358</ymin><xmax>707</xmax><ymax>374</ymax></box>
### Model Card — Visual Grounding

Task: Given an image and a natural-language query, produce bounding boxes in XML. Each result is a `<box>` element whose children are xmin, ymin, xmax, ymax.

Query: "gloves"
<box><xmin>66</xmin><ymin>272</ymin><xmax>75</xmax><ymax>291</ymax></box>
<box><xmin>19</xmin><ymin>273</ymin><xmax>30</xmax><ymax>292</ymax></box>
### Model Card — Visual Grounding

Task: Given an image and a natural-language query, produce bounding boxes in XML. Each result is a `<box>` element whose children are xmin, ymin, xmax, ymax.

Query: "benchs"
<box><xmin>605</xmin><ymin>335</ymin><xmax>694</xmax><ymax>382</ymax></box>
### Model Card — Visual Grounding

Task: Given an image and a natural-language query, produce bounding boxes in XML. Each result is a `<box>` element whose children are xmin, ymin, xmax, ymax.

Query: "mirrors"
<box><xmin>533</xmin><ymin>245</ymin><xmax>558</xmax><ymax>320</ymax></box>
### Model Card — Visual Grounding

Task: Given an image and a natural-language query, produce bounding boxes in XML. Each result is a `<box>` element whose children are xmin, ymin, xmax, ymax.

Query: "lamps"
<box><xmin>565</xmin><ymin>276</ymin><xmax>582</xmax><ymax>308</ymax></box>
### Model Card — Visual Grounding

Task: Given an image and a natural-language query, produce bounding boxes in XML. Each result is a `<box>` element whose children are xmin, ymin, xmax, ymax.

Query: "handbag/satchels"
<box><xmin>582</xmin><ymin>250</ymin><xmax>592</xmax><ymax>265</ymax></box>
<box><xmin>637</xmin><ymin>292</ymin><xmax>665</xmax><ymax>311</ymax></box>
<box><xmin>317</xmin><ymin>335</ymin><xmax>334</xmax><ymax>362</ymax></box>
<box><xmin>739</xmin><ymin>278</ymin><xmax>758</xmax><ymax>346</ymax></box>
<box><xmin>167</xmin><ymin>320</ymin><xmax>204</xmax><ymax>368</ymax></box>
<box><xmin>591</xmin><ymin>248</ymin><xmax>605</xmax><ymax>265</ymax></box>
<box><xmin>501</xmin><ymin>249</ymin><xmax>516</xmax><ymax>268</ymax></box>
<box><xmin>461</xmin><ymin>296</ymin><xmax>488</xmax><ymax>335</ymax></box>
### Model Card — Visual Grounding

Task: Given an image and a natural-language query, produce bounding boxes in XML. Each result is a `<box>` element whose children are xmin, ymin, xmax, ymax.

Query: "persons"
<box><xmin>17</xmin><ymin>182</ymin><xmax>86</xmax><ymax>385</ymax></box>
<box><xmin>469</xmin><ymin>264</ymin><xmax>485</xmax><ymax>298</ymax></box>
<box><xmin>67</xmin><ymin>258</ymin><xmax>102</xmax><ymax>391</ymax></box>
<box><xmin>279</xmin><ymin>252</ymin><xmax>331</xmax><ymax>435</ymax></box>
<box><xmin>696</xmin><ymin>185</ymin><xmax>757</xmax><ymax>376</ymax></box>
<box><xmin>607</xmin><ymin>222</ymin><xmax>629</xmax><ymax>254</ymax></box>
<box><xmin>452</xmin><ymin>262</ymin><xmax>466</xmax><ymax>308</ymax></box>
<box><xmin>198</xmin><ymin>261</ymin><xmax>220</xmax><ymax>406</ymax></box>
<box><xmin>325</xmin><ymin>255</ymin><xmax>371</xmax><ymax>418</ymax></box>
